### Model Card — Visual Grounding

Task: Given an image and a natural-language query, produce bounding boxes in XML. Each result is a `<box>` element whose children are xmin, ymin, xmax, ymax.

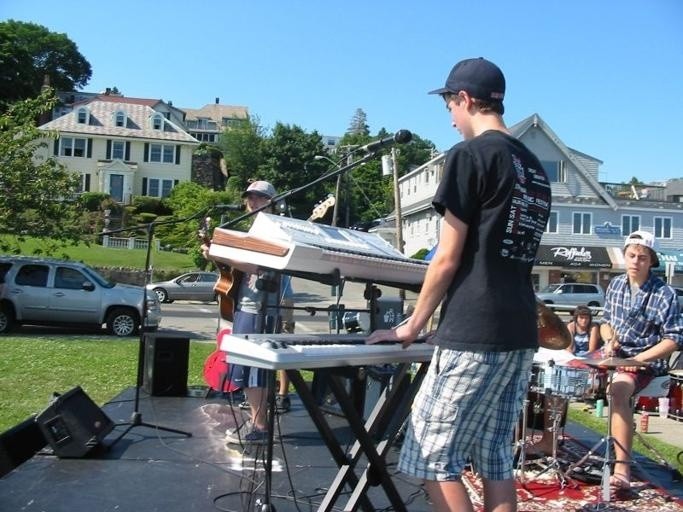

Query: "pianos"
<box><xmin>220</xmin><ymin>333</ymin><xmax>435</xmax><ymax>369</ymax></box>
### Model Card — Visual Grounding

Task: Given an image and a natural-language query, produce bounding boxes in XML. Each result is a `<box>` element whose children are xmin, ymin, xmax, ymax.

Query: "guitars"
<box><xmin>199</xmin><ymin>217</ymin><xmax>244</xmax><ymax>324</ymax></box>
<box><xmin>203</xmin><ymin>328</ymin><xmax>240</xmax><ymax>394</ymax></box>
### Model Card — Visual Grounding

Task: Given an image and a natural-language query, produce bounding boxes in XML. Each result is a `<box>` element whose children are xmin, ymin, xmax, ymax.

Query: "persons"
<box><xmin>523</xmin><ymin>230</ymin><xmax>683</xmax><ymax>491</ymax></box>
<box><xmin>365</xmin><ymin>58</ymin><xmax>552</xmax><ymax>511</ymax></box>
<box><xmin>201</xmin><ymin>182</ymin><xmax>278</xmax><ymax>446</ymax></box>
<box><xmin>237</xmin><ymin>278</ymin><xmax>294</xmax><ymax>415</ymax></box>
<box><xmin>566</xmin><ymin>306</ymin><xmax>599</xmax><ymax>355</ymax></box>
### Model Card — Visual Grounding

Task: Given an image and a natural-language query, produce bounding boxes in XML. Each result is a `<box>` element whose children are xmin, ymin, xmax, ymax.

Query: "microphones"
<box><xmin>358</xmin><ymin>129</ymin><xmax>413</xmax><ymax>152</ymax></box>
<box><xmin>209</xmin><ymin>204</ymin><xmax>246</xmax><ymax>212</ymax></box>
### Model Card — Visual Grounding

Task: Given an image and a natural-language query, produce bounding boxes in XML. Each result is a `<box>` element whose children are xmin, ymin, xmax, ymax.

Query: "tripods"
<box><xmin>530</xmin><ymin>372</ymin><xmax>582</xmax><ymax>490</ymax></box>
<box><xmin>513</xmin><ymin>376</ymin><xmax>552</xmax><ymax>484</ymax></box>
<box><xmin>569</xmin><ymin>377</ymin><xmax>673</xmax><ymax>508</ymax></box>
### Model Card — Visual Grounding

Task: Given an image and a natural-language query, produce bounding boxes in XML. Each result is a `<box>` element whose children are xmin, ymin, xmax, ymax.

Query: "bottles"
<box><xmin>595</xmin><ymin>399</ymin><xmax>604</xmax><ymax>418</ymax></box>
<box><xmin>638</xmin><ymin>406</ymin><xmax>650</xmax><ymax>434</ymax></box>
<box><xmin>384</xmin><ymin>308</ymin><xmax>401</xmax><ymax>330</ymax></box>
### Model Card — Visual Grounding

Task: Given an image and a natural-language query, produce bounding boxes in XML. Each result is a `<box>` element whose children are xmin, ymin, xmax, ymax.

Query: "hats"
<box><xmin>427</xmin><ymin>56</ymin><xmax>507</xmax><ymax>104</ymax></box>
<box><xmin>623</xmin><ymin>229</ymin><xmax>660</xmax><ymax>269</ymax></box>
<box><xmin>241</xmin><ymin>180</ymin><xmax>277</xmax><ymax>203</ymax></box>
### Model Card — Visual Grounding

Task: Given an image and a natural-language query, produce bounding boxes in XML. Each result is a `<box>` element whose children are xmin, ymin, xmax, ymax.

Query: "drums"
<box><xmin>669</xmin><ymin>369</ymin><xmax>683</xmax><ymax>421</ymax></box>
<box><xmin>530</xmin><ymin>362</ymin><xmax>591</xmax><ymax>400</ymax></box>
<box><xmin>633</xmin><ymin>397</ymin><xmax>671</xmax><ymax>419</ymax></box>
<box><xmin>342</xmin><ymin>308</ymin><xmax>369</xmax><ymax>332</ymax></box>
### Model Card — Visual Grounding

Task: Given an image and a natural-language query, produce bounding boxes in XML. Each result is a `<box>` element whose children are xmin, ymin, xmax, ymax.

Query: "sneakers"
<box><xmin>225</xmin><ymin>423</ymin><xmax>273</xmax><ymax>445</ymax></box>
<box><xmin>270</xmin><ymin>394</ymin><xmax>291</xmax><ymax>415</ymax></box>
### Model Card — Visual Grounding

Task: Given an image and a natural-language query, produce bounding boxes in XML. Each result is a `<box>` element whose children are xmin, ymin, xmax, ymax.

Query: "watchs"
<box><xmin>604</xmin><ymin>339</ymin><xmax>612</xmax><ymax>346</ymax></box>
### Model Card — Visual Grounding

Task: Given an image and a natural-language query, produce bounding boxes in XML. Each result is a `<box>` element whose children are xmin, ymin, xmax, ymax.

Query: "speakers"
<box><xmin>94</xmin><ymin>215</ymin><xmax>199</xmax><ymax>454</ymax></box>
<box><xmin>310</xmin><ymin>367</ymin><xmax>411</xmax><ymax>423</ymax></box>
<box><xmin>140</xmin><ymin>332</ymin><xmax>189</xmax><ymax>396</ymax></box>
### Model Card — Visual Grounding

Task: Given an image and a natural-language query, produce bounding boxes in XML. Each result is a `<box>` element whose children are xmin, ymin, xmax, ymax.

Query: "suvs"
<box><xmin>533</xmin><ymin>283</ymin><xmax>605</xmax><ymax>316</ymax></box>
<box><xmin>0</xmin><ymin>258</ymin><xmax>161</xmax><ymax>337</ymax></box>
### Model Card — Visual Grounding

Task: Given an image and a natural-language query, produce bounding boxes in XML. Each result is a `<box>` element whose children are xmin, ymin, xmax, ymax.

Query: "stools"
<box><xmin>601</xmin><ymin>370</ymin><xmax>672</xmax><ymax>476</ymax></box>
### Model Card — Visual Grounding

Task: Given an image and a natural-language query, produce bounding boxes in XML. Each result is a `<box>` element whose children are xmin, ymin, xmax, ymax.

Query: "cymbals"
<box><xmin>582</xmin><ymin>357</ymin><xmax>650</xmax><ymax>367</ymax></box>
<box><xmin>536</xmin><ymin>298</ymin><xmax>571</xmax><ymax>350</ymax></box>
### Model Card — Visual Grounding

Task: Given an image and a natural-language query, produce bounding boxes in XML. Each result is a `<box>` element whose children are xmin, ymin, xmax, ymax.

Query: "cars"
<box><xmin>141</xmin><ymin>272</ymin><xmax>220</xmax><ymax>304</ymax></box>
<box><xmin>670</xmin><ymin>286</ymin><xmax>682</xmax><ymax>314</ymax></box>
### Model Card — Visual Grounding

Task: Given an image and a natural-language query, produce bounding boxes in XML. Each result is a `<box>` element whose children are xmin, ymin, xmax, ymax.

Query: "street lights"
<box><xmin>313</xmin><ymin>146</ymin><xmax>366</xmax><ymax>230</ymax></box>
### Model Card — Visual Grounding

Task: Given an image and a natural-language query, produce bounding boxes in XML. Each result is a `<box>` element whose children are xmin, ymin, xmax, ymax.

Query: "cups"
<box><xmin>657</xmin><ymin>394</ymin><xmax>669</xmax><ymax>419</ymax></box>
